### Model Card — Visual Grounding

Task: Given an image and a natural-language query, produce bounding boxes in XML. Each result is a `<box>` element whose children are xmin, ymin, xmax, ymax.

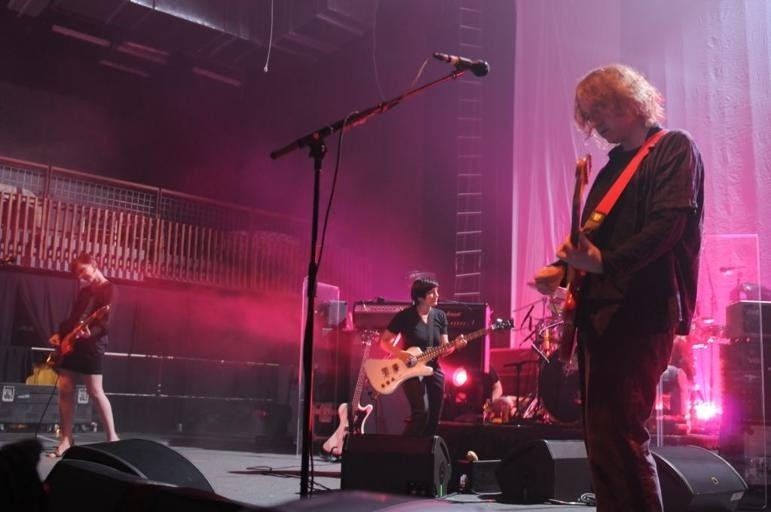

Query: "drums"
<box><xmin>536</xmin><ymin>347</ymin><xmax>583</xmax><ymax>424</ymax></box>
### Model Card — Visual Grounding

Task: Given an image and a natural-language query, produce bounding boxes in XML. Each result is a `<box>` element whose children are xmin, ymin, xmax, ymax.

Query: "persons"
<box><xmin>527</xmin><ymin>63</ymin><xmax>707</xmax><ymax>509</ymax></box>
<box><xmin>26</xmin><ymin>349</ymin><xmax>62</xmax><ymax>386</ymax></box>
<box><xmin>450</xmin><ymin>364</ymin><xmax>504</xmax><ymax>469</ymax></box>
<box><xmin>661</xmin><ymin>289</ymin><xmax>715</xmax><ymax>435</ymax></box>
<box><xmin>380</xmin><ymin>277</ymin><xmax>470</xmax><ymax>438</ymax></box>
<box><xmin>46</xmin><ymin>253</ymin><xmax>121</xmax><ymax>457</ymax></box>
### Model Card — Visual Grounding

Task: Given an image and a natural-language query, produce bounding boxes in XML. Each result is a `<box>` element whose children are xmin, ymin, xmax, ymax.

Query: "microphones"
<box><xmin>433</xmin><ymin>52</ymin><xmax>489</xmax><ymax>77</ymax></box>
<box><xmin>721</xmin><ymin>266</ymin><xmax>745</xmax><ymax>271</ymax></box>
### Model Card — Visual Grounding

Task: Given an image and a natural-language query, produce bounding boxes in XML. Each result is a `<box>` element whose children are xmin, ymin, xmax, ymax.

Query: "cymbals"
<box><xmin>526</xmin><ymin>283</ymin><xmax>567</xmax><ymax>297</ymax></box>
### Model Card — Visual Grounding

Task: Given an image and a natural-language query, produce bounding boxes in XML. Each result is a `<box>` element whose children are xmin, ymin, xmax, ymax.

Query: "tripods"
<box><xmin>500</xmin><ymin>296</ymin><xmax>565</xmax><ymax>425</ymax></box>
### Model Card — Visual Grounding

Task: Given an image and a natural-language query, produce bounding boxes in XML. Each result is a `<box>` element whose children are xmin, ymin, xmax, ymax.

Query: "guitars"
<box><xmin>558</xmin><ymin>152</ymin><xmax>591</xmax><ymax>376</ymax></box>
<box><xmin>321</xmin><ymin>328</ymin><xmax>376</xmax><ymax>455</ymax></box>
<box><xmin>46</xmin><ymin>305</ymin><xmax>110</xmax><ymax>368</ymax></box>
<box><xmin>365</xmin><ymin>319</ymin><xmax>513</xmax><ymax>396</ymax></box>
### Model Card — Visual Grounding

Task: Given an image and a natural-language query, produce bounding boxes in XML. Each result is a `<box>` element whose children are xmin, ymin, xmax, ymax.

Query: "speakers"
<box><xmin>60</xmin><ymin>437</ymin><xmax>218</xmax><ymax>494</ymax></box>
<box><xmin>649</xmin><ymin>444</ymin><xmax>749</xmax><ymax>512</ymax></box>
<box><xmin>340</xmin><ymin>433</ymin><xmax>452</xmax><ymax>499</ymax></box>
<box><xmin>493</xmin><ymin>439</ymin><xmax>592</xmax><ymax>502</ymax></box>
